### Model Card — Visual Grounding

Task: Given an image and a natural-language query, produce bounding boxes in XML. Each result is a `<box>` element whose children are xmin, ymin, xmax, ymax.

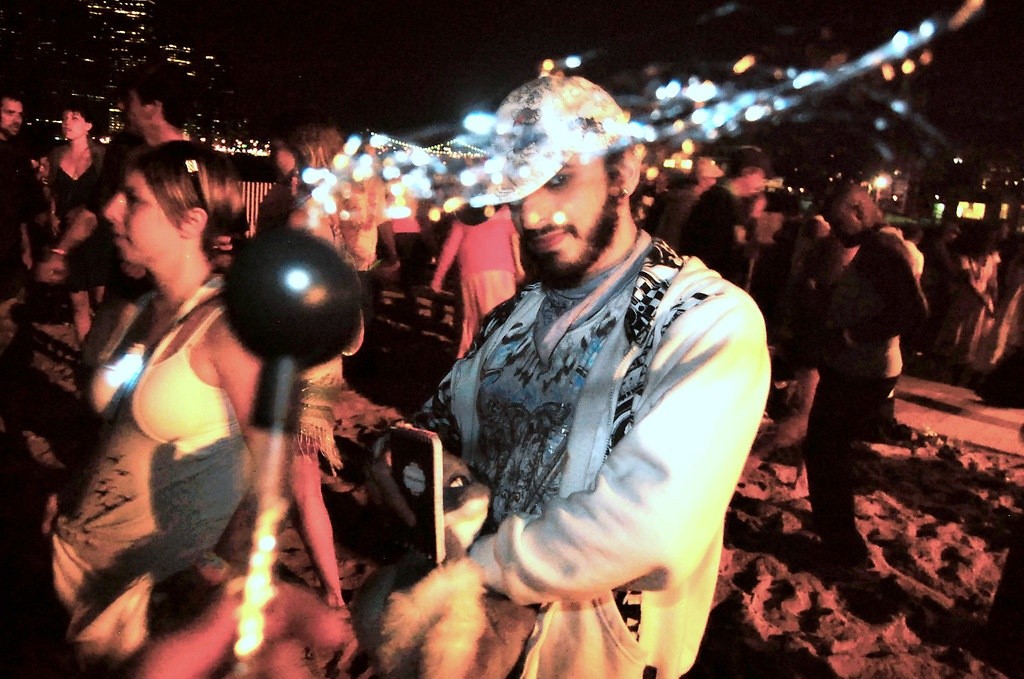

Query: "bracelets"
<box><xmin>50</xmin><ymin>248</ymin><xmax>69</xmax><ymax>257</ymax></box>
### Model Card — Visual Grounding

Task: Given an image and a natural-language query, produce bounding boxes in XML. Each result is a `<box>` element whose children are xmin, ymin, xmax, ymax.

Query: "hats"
<box><xmin>459</xmin><ymin>74</ymin><xmax>635</xmax><ymax>205</ymax></box>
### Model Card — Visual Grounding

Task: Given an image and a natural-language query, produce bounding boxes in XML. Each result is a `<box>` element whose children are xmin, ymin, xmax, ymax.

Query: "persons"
<box><xmin>0</xmin><ymin>68</ymin><xmax>1024</xmax><ymax>564</ymax></box>
<box><xmin>40</xmin><ymin>141</ymin><xmax>364</xmax><ymax>679</ymax></box>
<box><xmin>376</xmin><ymin>76</ymin><xmax>771</xmax><ymax>679</ymax></box>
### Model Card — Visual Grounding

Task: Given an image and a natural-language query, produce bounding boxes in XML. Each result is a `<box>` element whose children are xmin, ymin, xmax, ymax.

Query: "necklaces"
<box><xmin>125</xmin><ymin>290</ymin><xmax>228</xmax><ymax>365</ymax></box>
